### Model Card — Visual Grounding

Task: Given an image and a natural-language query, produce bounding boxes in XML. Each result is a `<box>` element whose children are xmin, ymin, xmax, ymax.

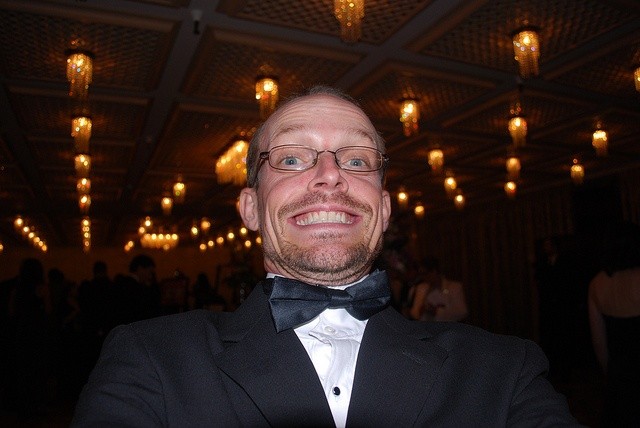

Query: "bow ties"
<box><xmin>267</xmin><ymin>267</ymin><xmax>392</xmax><ymax>334</ymax></box>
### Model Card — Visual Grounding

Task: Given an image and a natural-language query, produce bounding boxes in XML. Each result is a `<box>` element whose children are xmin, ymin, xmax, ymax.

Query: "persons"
<box><xmin>69</xmin><ymin>86</ymin><xmax>579</xmax><ymax>428</ymax></box>
<box><xmin>589</xmin><ymin>218</ymin><xmax>640</xmax><ymax>389</ymax></box>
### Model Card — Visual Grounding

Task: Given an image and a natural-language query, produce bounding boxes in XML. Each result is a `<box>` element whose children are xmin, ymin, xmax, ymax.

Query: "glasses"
<box><xmin>250</xmin><ymin>143</ymin><xmax>390</xmax><ymax>190</ymax></box>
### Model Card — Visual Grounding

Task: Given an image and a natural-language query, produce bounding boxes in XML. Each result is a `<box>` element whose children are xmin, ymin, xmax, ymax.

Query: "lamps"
<box><xmin>632</xmin><ymin>63</ymin><xmax>640</xmax><ymax>94</ymax></box>
<box><xmin>590</xmin><ymin>123</ymin><xmax>611</xmax><ymax>159</ymax></box>
<box><xmin>69</xmin><ymin>112</ymin><xmax>94</xmax><ymax>153</ymax></box>
<box><xmin>63</xmin><ymin>43</ymin><xmax>96</xmax><ymax>101</ymax></box>
<box><xmin>255</xmin><ymin>74</ymin><xmax>281</xmax><ymax>119</ymax></box>
<box><xmin>508</xmin><ymin>24</ymin><xmax>544</xmax><ymax>81</ymax></box>
<box><xmin>397</xmin><ymin>96</ymin><xmax>422</xmax><ymax>138</ymax></box>
<box><xmin>506</xmin><ymin>113</ymin><xmax>529</xmax><ymax>148</ymax></box>
